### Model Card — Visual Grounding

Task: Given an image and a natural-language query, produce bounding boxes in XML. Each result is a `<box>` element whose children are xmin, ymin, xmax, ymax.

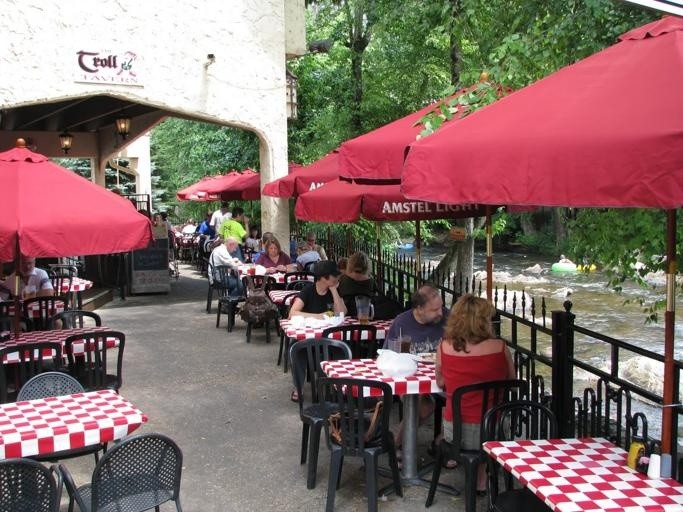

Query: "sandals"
<box><xmin>426</xmin><ymin>437</ymin><xmax>458</xmax><ymax>469</ymax></box>
<box><xmin>290</xmin><ymin>390</ymin><xmax>303</xmax><ymax>402</ymax></box>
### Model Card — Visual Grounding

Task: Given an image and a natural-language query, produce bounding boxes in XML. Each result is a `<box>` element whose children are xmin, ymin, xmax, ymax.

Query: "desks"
<box><xmin>0</xmin><ymin>390</ymin><xmax>149</xmax><ymax>467</ymax></box>
<box><xmin>321</xmin><ymin>353</ymin><xmax>459</xmax><ymax>497</ymax></box>
<box><xmin>483</xmin><ymin>437</ymin><xmax>683</xmax><ymax>511</ymax></box>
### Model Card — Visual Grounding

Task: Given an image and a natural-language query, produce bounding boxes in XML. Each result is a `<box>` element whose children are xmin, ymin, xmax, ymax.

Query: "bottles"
<box><xmin>247</xmin><ymin>268</ymin><xmax>255</xmax><ymax>276</ymax></box>
<box><xmin>626</xmin><ymin>435</ymin><xmax>660</xmax><ymax>479</ymax></box>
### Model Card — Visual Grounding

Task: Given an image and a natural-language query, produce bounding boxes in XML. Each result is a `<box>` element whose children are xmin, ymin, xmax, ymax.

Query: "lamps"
<box><xmin>112</xmin><ymin>105</ymin><xmax>134</xmax><ymax>141</ymax></box>
<box><xmin>58</xmin><ymin>127</ymin><xmax>73</xmax><ymax>157</ymax></box>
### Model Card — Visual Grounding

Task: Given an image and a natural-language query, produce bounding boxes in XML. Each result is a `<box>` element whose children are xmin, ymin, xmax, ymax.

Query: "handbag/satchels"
<box><xmin>327</xmin><ymin>400</ymin><xmax>386</xmax><ymax>448</ymax></box>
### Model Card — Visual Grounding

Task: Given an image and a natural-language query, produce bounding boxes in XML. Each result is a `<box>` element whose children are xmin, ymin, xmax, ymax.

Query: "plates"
<box><xmin>419</xmin><ymin>353</ymin><xmax>438</xmax><ymax>363</ymax></box>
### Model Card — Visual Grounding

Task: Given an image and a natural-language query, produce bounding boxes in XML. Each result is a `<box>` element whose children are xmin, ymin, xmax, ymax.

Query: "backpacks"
<box><xmin>240</xmin><ymin>274</ymin><xmax>275</xmax><ymax>325</ymax></box>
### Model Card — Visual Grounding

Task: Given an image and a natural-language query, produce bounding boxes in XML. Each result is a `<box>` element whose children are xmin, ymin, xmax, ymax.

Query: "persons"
<box><xmin>2</xmin><ymin>253</ymin><xmax>62</xmax><ymax>330</ymax></box>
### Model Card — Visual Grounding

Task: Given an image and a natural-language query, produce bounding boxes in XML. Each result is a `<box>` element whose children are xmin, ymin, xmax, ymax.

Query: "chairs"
<box><xmin>65</xmin><ymin>331</ymin><xmax>125</xmax><ymax>391</ymax></box>
<box><xmin>288</xmin><ymin>338</ymin><xmax>372</xmax><ymax>491</ymax></box>
<box><xmin>0</xmin><ymin>262</ymin><xmax>120</xmax><ymax>383</ymax></box>
<box><xmin>317</xmin><ymin>377</ymin><xmax>404</xmax><ymax>511</ymax></box>
<box><xmin>397</xmin><ymin>394</ymin><xmax>446</xmax><ymax>435</ymax></box>
<box><xmin>58</xmin><ymin>433</ymin><xmax>183</xmax><ymax>511</ymax></box>
<box><xmin>171</xmin><ymin>218</ymin><xmax>450</xmax><ymax>343</ymax></box>
<box><xmin>426</xmin><ymin>379</ymin><xmax>529</xmax><ymax>511</ymax></box>
<box><xmin>483</xmin><ymin>402</ymin><xmax>557</xmax><ymax>511</ymax></box>
<box><xmin>1</xmin><ymin>458</ymin><xmax>63</xmax><ymax>511</ymax></box>
<box><xmin>2</xmin><ymin>342</ymin><xmax>60</xmax><ymax>403</ymax></box>
<box><xmin>17</xmin><ymin>373</ymin><xmax>106</xmax><ymax>510</ymax></box>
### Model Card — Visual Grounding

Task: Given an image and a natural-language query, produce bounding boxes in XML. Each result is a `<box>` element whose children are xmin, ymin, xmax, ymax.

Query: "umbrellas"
<box><xmin>0</xmin><ymin>137</ymin><xmax>153</xmax><ymax>350</ymax></box>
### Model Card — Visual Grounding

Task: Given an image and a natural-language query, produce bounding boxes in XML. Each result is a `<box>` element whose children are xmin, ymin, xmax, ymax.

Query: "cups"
<box><xmin>388</xmin><ymin>337</ymin><xmax>401</xmax><ymax>354</ymax></box>
<box><xmin>398</xmin><ymin>335</ymin><xmax>411</xmax><ymax>353</ymax></box>
<box><xmin>355</xmin><ymin>296</ymin><xmax>374</xmax><ymax>325</ymax></box>
<box><xmin>21</xmin><ymin>285</ymin><xmax>37</xmax><ymax>299</ymax></box>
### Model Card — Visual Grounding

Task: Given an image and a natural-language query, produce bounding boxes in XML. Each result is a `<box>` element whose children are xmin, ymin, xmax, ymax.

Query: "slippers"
<box><xmin>475</xmin><ymin>487</ymin><xmax>488</xmax><ymax>497</ymax></box>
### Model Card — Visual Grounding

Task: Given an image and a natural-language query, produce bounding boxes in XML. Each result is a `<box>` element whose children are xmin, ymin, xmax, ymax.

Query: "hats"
<box><xmin>313</xmin><ymin>261</ymin><xmax>342</xmax><ymax>276</ymax></box>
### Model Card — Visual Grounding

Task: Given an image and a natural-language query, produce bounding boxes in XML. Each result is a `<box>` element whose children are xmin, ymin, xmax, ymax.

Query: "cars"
<box><xmin>171</xmin><ymin>223</ymin><xmax>203</xmax><ymax>259</ymax></box>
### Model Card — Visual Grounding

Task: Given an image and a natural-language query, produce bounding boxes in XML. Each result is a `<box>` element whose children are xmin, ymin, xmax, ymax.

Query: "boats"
<box><xmin>400</xmin><ymin>244</ymin><xmax>413</xmax><ymax>250</ymax></box>
<box><xmin>553</xmin><ymin>263</ymin><xmax>597</xmax><ymax>273</ymax></box>
